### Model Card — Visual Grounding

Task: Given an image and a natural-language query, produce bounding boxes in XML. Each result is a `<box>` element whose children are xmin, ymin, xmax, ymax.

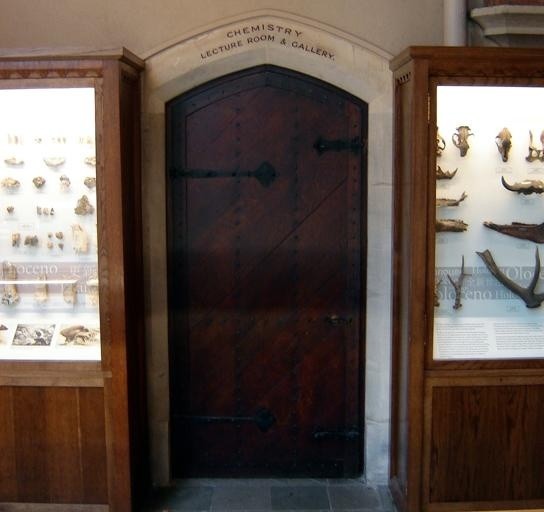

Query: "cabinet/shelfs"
<box><xmin>0</xmin><ymin>44</ymin><xmax>151</xmax><ymax>512</ymax></box>
<box><xmin>386</xmin><ymin>44</ymin><xmax>544</xmax><ymax>511</ymax></box>
